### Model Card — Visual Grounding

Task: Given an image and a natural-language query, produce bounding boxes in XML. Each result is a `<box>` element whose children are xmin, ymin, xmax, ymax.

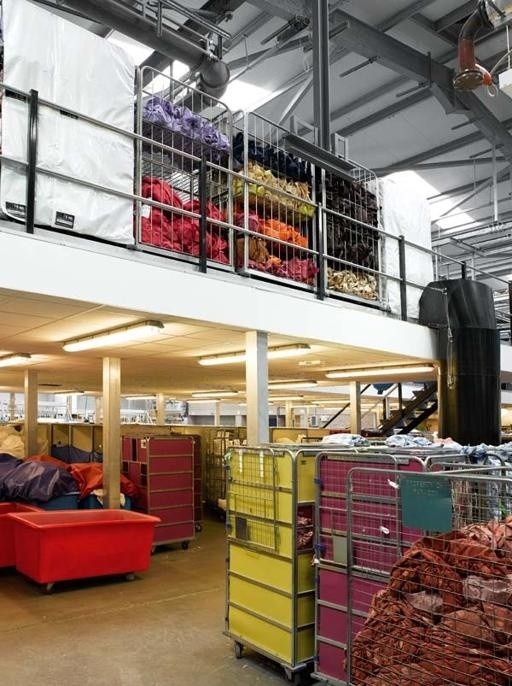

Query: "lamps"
<box><xmin>190</xmin><ymin>390</ymin><xmax>239</xmax><ymax>399</ymax></box>
<box><xmin>196</xmin><ymin>342</ymin><xmax>312</xmax><ymax>367</ymax></box>
<box><xmin>0</xmin><ymin>352</ymin><xmax>32</xmax><ymax>368</ymax></box>
<box><xmin>324</xmin><ymin>363</ymin><xmax>435</xmax><ymax>380</ymax></box>
<box><xmin>268</xmin><ymin>380</ymin><xmax>317</xmax><ymax>389</ymax></box>
<box><xmin>61</xmin><ymin>320</ymin><xmax>165</xmax><ymax>353</ymax></box>
